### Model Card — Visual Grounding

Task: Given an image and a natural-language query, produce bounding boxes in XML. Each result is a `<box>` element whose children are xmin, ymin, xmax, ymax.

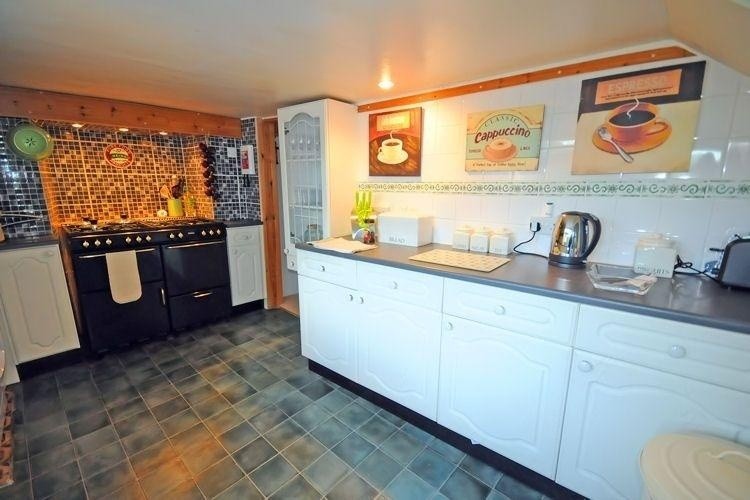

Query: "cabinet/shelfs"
<box><xmin>297</xmin><ymin>247</ymin><xmax>440</xmax><ymax>425</ymax></box>
<box><xmin>74</xmin><ymin>240</ymin><xmax>263</xmax><ymax>355</ymax></box>
<box><xmin>225</xmin><ymin>224</ymin><xmax>267</xmax><ymax>307</ymax></box>
<box><xmin>555</xmin><ymin>301</ymin><xmax>750</xmax><ymax>495</ymax></box>
<box><xmin>1</xmin><ymin>244</ymin><xmax>82</xmax><ymax>389</ymax></box>
<box><xmin>278</xmin><ymin>98</ymin><xmax>358</xmax><ymax>275</ymax></box>
<box><xmin>434</xmin><ymin>277</ymin><xmax>583</xmax><ymax>484</ymax></box>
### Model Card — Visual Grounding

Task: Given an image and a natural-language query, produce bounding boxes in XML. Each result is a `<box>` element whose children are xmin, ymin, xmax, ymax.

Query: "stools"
<box><xmin>638</xmin><ymin>430</ymin><xmax>750</xmax><ymax>495</ymax></box>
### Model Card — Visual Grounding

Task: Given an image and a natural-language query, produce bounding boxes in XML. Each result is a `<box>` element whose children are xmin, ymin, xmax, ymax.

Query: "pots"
<box><xmin>6</xmin><ymin>119</ymin><xmax>54</xmax><ymax>161</ymax></box>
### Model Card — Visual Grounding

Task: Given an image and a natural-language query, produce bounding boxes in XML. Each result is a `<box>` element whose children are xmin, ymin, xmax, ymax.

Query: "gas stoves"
<box><xmin>61</xmin><ymin>217</ymin><xmax>225</xmax><ymax>257</ymax></box>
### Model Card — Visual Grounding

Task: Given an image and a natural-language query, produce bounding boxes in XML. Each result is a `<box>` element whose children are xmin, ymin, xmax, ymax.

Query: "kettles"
<box><xmin>546</xmin><ymin>209</ymin><xmax>602</xmax><ymax>270</ymax></box>
